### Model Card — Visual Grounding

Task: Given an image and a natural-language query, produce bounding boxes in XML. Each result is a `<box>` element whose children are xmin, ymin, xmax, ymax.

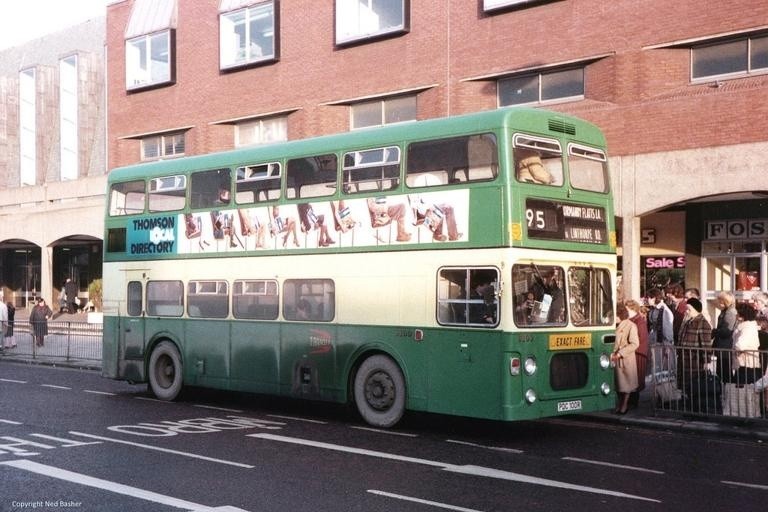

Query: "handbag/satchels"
<box><xmin>687</xmin><ymin>375</ymin><xmax>719</xmax><ymax>414</ymax></box>
<box><xmin>720</xmin><ymin>383</ymin><xmax>760</xmax><ymax>417</ymax></box>
<box><xmin>653</xmin><ymin>369</ymin><xmax>681</xmax><ymax>403</ymax></box>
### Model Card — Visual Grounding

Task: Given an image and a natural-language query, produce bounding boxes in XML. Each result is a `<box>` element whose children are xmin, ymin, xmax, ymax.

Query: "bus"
<box><xmin>96</xmin><ymin>107</ymin><xmax>619</xmax><ymax>427</ymax></box>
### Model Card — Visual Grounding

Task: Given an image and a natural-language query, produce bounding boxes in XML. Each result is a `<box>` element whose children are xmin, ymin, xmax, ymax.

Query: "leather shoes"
<box><xmin>614</xmin><ymin>407</ymin><xmax>628</xmax><ymax>415</ymax></box>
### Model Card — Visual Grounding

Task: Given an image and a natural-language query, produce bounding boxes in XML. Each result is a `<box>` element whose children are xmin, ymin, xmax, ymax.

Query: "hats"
<box><xmin>687</xmin><ymin>298</ymin><xmax>702</xmax><ymax>313</ymax></box>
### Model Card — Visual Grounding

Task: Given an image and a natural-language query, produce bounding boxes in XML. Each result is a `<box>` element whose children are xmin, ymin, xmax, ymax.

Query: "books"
<box><xmin>421</xmin><ymin>204</ymin><xmax>445</xmax><ymax>233</ymax></box>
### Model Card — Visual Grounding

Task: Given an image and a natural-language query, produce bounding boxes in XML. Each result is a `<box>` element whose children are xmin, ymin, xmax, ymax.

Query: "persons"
<box><xmin>210</xmin><ymin>210</ymin><xmax>238</xmax><ymax>249</ymax></box>
<box><xmin>272</xmin><ymin>206</ymin><xmax>302</xmax><ymax>250</ymax></box>
<box><xmin>329</xmin><ymin>200</ymin><xmax>363</xmax><ymax>234</ymax></box>
<box><xmin>4</xmin><ymin>302</ymin><xmax>19</xmax><ymax>350</ymax></box>
<box><xmin>28</xmin><ymin>297</ymin><xmax>52</xmax><ymax>347</ymax></box>
<box><xmin>0</xmin><ymin>296</ymin><xmax>8</xmax><ymax>351</ymax></box>
<box><xmin>183</xmin><ymin>213</ymin><xmax>210</xmax><ymax>252</ymax></box>
<box><xmin>236</xmin><ymin>208</ymin><xmax>268</xmax><ymax>250</ymax></box>
<box><xmin>365</xmin><ymin>196</ymin><xmax>414</xmax><ymax>244</ymax></box>
<box><xmin>407</xmin><ymin>193</ymin><xmax>464</xmax><ymax>242</ymax></box>
<box><xmin>57</xmin><ymin>286</ymin><xmax>68</xmax><ymax>312</ymax></box>
<box><xmin>452</xmin><ymin>266</ymin><xmax>768</xmax><ymax>428</ymax></box>
<box><xmin>213</xmin><ymin>188</ymin><xmax>231</xmax><ymax>204</ymax></box>
<box><xmin>293</xmin><ymin>299</ymin><xmax>313</xmax><ymax>322</ymax></box>
<box><xmin>64</xmin><ymin>278</ymin><xmax>78</xmax><ymax>314</ymax></box>
<box><xmin>297</xmin><ymin>204</ymin><xmax>336</xmax><ymax>248</ymax></box>
<box><xmin>515</xmin><ymin>140</ymin><xmax>558</xmax><ymax>186</ymax></box>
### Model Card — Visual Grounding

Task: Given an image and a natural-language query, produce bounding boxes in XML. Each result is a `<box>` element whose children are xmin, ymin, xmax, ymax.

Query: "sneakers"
<box><xmin>433</xmin><ymin>233</ymin><xmax>448</xmax><ymax>242</ymax></box>
<box><xmin>230</xmin><ymin>241</ymin><xmax>237</xmax><ymax>248</ymax></box>
<box><xmin>451</xmin><ymin>234</ymin><xmax>464</xmax><ymax>241</ymax></box>
<box><xmin>320</xmin><ymin>239</ymin><xmax>335</xmax><ymax>247</ymax></box>
<box><xmin>282</xmin><ymin>234</ymin><xmax>288</xmax><ymax>247</ymax></box>
<box><xmin>256</xmin><ymin>243</ymin><xmax>263</xmax><ymax>248</ymax></box>
<box><xmin>198</xmin><ymin>241</ymin><xmax>209</xmax><ymax>250</ymax></box>
<box><xmin>397</xmin><ymin>231</ymin><xmax>412</xmax><ymax>241</ymax></box>
<box><xmin>293</xmin><ymin>240</ymin><xmax>299</xmax><ymax>246</ymax></box>
